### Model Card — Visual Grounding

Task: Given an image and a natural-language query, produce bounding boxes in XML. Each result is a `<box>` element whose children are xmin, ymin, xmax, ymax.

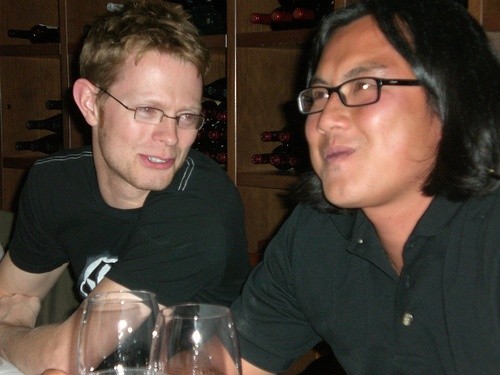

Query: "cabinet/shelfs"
<box><xmin>0</xmin><ymin>0</ymin><xmax>499</xmax><ymax>375</ymax></box>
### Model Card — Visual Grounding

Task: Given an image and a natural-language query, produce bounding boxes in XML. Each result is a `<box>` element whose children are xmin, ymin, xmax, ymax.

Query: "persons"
<box><xmin>0</xmin><ymin>0</ymin><xmax>253</xmax><ymax>375</ymax></box>
<box><xmin>168</xmin><ymin>0</ymin><xmax>500</xmax><ymax>375</ymax></box>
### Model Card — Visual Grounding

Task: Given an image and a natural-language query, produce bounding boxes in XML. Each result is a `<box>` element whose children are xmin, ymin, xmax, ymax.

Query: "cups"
<box><xmin>147</xmin><ymin>303</ymin><xmax>242</xmax><ymax>375</ymax></box>
<box><xmin>76</xmin><ymin>291</ymin><xmax>165</xmax><ymax>375</ymax></box>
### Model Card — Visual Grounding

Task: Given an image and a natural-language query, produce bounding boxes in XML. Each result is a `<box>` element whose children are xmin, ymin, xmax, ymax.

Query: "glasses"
<box><xmin>96</xmin><ymin>86</ymin><xmax>205</xmax><ymax>131</ymax></box>
<box><xmin>298</xmin><ymin>77</ymin><xmax>425</xmax><ymax>114</ymax></box>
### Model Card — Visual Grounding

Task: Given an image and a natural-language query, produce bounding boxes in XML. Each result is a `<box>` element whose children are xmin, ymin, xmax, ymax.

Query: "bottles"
<box><xmin>107</xmin><ymin>3</ymin><xmax>128</xmax><ymax>14</ymax></box>
<box><xmin>166</xmin><ymin>0</ymin><xmax>226</xmax><ymax>35</ymax></box>
<box><xmin>83</xmin><ymin>25</ymin><xmax>92</xmax><ymax>32</ymax></box>
<box><xmin>190</xmin><ymin>76</ymin><xmax>227</xmax><ymax>165</ymax></box>
<box><xmin>249</xmin><ymin>0</ymin><xmax>334</xmax><ymax>32</ymax></box>
<box><xmin>15</xmin><ymin>99</ymin><xmax>64</xmax><ymax>156</ymax></box>
<box><xmin>7</xmin><ymin>24</ymin><xmax>60</xmax><ymax>44</ymax></box>
<box><xmin>250</xmin><ymin>124</ymin><xmax>311</xmax><ymax>172</ymax></box>
